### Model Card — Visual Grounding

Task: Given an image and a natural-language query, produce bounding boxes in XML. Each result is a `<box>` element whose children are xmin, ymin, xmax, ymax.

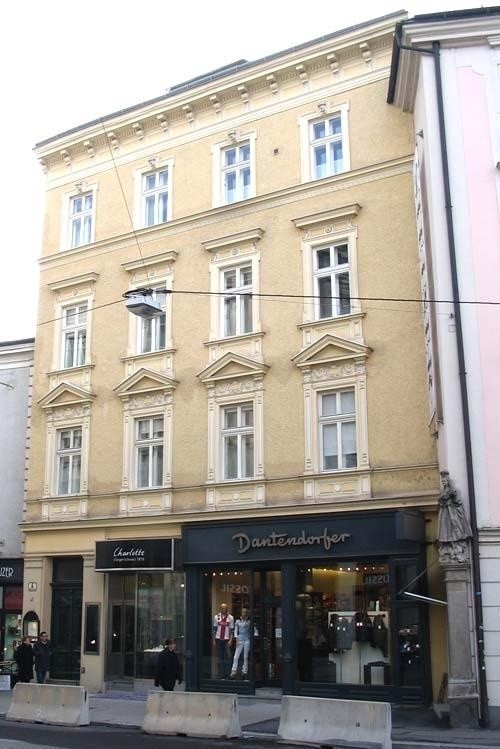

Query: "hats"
<box><xmin>165</xmin><ymin>639</ymin><xmax>176</xmax><ymax>645</ymax></box>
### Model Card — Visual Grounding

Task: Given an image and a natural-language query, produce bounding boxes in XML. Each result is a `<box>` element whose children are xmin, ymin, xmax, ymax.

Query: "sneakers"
<box><xmin>226</xmin><ymin>674</ymin><xmax>250</xmax><ymax>682</ymax></box>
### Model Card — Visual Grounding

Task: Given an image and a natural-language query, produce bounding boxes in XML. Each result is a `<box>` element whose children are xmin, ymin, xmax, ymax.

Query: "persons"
<box><xmin>227</xmin><ymin>607</ymin><xmax>259</xmax><ymax>680</ymax></box>
<box><xmin>154</xmin><ymin>638</ymin><xmax>183</xmax><ymax>691</ymax></box>
<box><xmin>212</xmin><ymin>603</ymin><xmax>235</xmax><ymax>677</ymax></box>
<box><xmin>14</xmin><ymin>636</ymin><xmax>33</xmax><ymax>682</ymax></box>
<box><xmin>31</xmin><ymin>632</ymin><xmax>53</xmax><ymax>682</ymax></box>
<box><xmin>438</xmin><ymin>468</ymin><xmax>471</xmax><ymax>563</ymax></box>
